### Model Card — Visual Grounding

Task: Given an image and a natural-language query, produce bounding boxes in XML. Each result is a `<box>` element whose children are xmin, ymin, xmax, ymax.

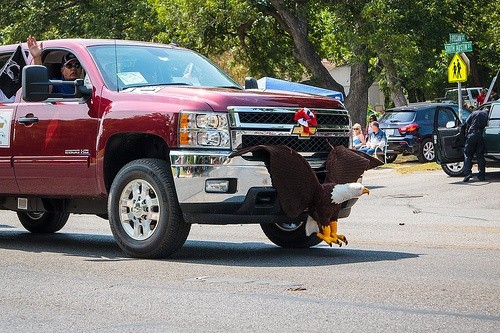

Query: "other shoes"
<box><xmin>476</xmin><ymin>173</ymin><xmax>485</xmax><ymax>181</ymax></box>
<box><xmin>463</xmin><ymin>173</ymin><xmax>472</xmax><ymax>182</ymax></box>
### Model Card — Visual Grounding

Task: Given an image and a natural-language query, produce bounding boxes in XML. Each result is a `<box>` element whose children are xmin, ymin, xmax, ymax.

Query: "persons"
<box><xmin>352</xmin><ymin>123</ymin><xmax>364</xmax><ymax>150</ymax></box>
<box><xmin>27</xmin><ymin>36</ymin><xmax>93</xmax><ymax>96</ymax></box>
<box><xmin>463</xmin><ymin>106</ymin><xmax>490</xmax><ymax>183</ymax></box>
<box><xmin>491</xmin><ymin>90</ymin><xmax>499</xmax><ymax>100</ymax></box>
<box><xmin>367</xmin><ymin>115</ymin><xmax>378</xmax><ymax>132</ymax></box>
<box><xmin>359</xmin><ymin>122</ymin><xmax>387</xmax><ymax>158</ymax></box>
<box><xmin>476</xmin><ymin>88</ymin><xmax>491</xmax><ymax>106</ymax></box>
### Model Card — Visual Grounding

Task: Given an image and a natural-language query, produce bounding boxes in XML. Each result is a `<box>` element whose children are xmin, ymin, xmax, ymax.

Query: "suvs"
<box><xmin>0</xmin><ymin>38</ymin><xmax>362</xmax><ymax>258</ymax></box>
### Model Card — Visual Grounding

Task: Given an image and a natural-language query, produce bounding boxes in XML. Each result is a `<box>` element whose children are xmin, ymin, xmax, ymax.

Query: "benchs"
<box><xmin>372</xmin><ymin>130</ymin><xmax>390</xmax><ymax>164</ymax></box>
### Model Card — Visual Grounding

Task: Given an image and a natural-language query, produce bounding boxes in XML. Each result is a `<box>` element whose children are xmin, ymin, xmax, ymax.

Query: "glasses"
<box><xmin>66</xmin><ymin>62</ymin><xmax>81</xmax><ymax>69</ymax></box>
<box><xmin>354</xmin><ymin>128</ymin><xmax>359</xmax><ymax>130</ymax></box>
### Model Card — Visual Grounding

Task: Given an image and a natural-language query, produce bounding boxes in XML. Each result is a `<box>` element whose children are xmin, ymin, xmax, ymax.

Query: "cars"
<box><xmin>377</xmin><ymin>87</ymin><xmax>500</xmax><ymax>177</ymax></box>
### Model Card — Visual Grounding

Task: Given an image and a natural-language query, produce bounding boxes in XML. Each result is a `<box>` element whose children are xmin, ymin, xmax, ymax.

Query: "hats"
<box><xmin>61</xmin><ymin>52</ymin><xmax>78</xmax><ymax>67</ymax></box>
